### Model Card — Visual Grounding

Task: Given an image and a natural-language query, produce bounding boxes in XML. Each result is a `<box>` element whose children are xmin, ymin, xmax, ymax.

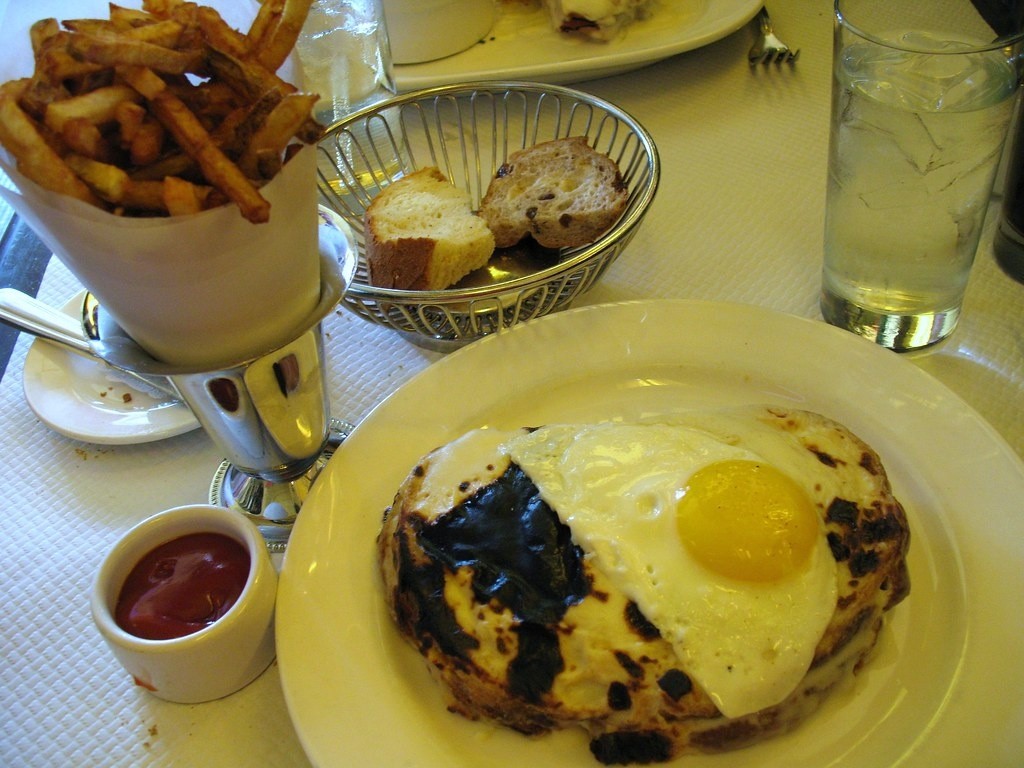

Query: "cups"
<box><xmin>257</xmin><ymin>0</ymin><xmax>411</xmax><ymax>217</ymax></box>
<box><xmin>820</xmin><ymin>0</ymin><xmax>1024</xmax><ymax>354</ymax></box>
<box><xmin>90</xmin><ymin>503</ymin><xmax>277</xmax><ymax>705</ymax></box>
<box><xmin>993</xmin><ymin>80</ymin><xmax>1024</xmax><ymax>285</ymax></box>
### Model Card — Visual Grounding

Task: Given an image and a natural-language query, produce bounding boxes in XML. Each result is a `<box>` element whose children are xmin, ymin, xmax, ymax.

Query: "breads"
<box><xmin>363</xmin><ymin>166</ymin><xmax>495</xmax><ymax>311</ymax></box>
<box><xmin>476</xmin><ymin>138</ymin><xmax>627</xmax><ymax>248</ymax></box>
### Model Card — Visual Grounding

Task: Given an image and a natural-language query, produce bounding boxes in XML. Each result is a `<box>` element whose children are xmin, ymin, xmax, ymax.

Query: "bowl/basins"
<box><xmin>380</xmin><ymin>0</ymin><xmax>497</xmax><ymax>65</ymax></box>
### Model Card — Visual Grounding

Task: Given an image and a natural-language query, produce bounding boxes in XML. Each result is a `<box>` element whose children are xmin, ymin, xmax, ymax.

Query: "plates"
<box><xmin>381</xmin><ymin>0</ymin><xmax>763</xmax><ymax>95</ymax></box>
<box><xmin>23</xmin><ymin>288</ymin><xmax>203</xmax><ymax>446</ymax></box>
<box><xmin>273</xmin><ymin>298</ymin><xmax>1024</xmax><ymax>768</ymax></box>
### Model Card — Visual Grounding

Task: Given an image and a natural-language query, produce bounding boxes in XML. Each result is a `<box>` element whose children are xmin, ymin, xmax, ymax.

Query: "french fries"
<box><xmin>0</xmin><ymin>0</ymin><xmax>324</xmax><ymax>224</ymax></box>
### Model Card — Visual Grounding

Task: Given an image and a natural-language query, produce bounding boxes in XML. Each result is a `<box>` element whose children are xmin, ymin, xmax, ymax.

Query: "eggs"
<box><xmin>517</xmin><ymin>428</ymin><xmax>838</xmax><ymax>716</ymax></box>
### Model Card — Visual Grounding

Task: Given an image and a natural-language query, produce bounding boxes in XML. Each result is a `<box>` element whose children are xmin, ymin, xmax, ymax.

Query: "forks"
<box><xmin>749</xmin><ymin>5</ymin><xmax>801</xmax><ymax>65</ymax></box>
